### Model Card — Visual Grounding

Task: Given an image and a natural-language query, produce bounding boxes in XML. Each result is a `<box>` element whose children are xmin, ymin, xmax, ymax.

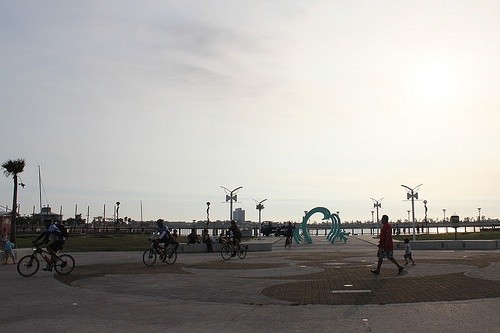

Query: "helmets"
<box><xmin>156</xmin><ymin>219</ymin><xmax>164</xmax><ymax>223</ymax></box>
<box><xmin>231</xmin><ymin>220</ymin><xmax>236</xmax><ymax>224</ymax></box>
<box><xmin>43</xmin><ymin>218</ymin><xmax>55</xmax><ymax>228</ymax></box>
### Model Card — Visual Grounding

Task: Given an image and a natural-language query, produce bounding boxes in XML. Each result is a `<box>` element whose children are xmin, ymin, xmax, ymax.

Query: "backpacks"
<box><xmin>57</xmin><ymin>224</ymin><xmax>70</xmax><ymax>240</ymax></box>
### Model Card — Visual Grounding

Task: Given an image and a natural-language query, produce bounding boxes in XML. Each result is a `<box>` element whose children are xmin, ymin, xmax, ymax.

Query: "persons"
<box><xmin>403</xmin><ymin>224</ymin><xmax>406</xmax><ymax>233</ymax></box>
<box><xmin>1</xmin><ymin>227</ymin><xmax>8</xmax><ymax>251</ymax></box>
<box><xmin>3</xmin><ymin>239</ymin><xmax>17</xmax><ymax>265</ymax></box>
<box><xmin>187</xmin><ymin>228</ymin><xmax>198</xmax><ymax>244</ymax></box>
<box><xmin>148</xmin><ymin>219</ymin><xmax>171</xmax><ymax>262</ymax></box>
<box><xmin>220</xmin><ymin>231</ymin><xmax>224</xmax><ymax>236</ymax></box>
<box><xmin>32</xmin><ymin>218</ymin><xmax>65</xmax><ymax>272</ymax></box>
<box><xmin>202</xmin><ymin>230</ymin><xmax>213</xmax><ymax>253</ymax></box>
<box><xmin>393</xmin><ymin>224</ymin><xmax>396</xmax><ymax>234</ymax></box>
<box><xmin>396</xmin><ymin>224</ymin><xmax>400</xmax><ymax>234</ymax></box>
<box><xmin>404</xmin><ymin>239</ymin><xmax>414</xmax><ymax>265</ymax></box>
<box><xmin>227</xmin><ymin>221</ymin><xmax>241</xmax><ymax>257</ymax></box>
<box><xmin>219</xmin><ymin>234</ymin><xmax>227</xmax><ymax>244</ymax></box>
<box><xmin>370</xmin><ymin>215</ymin><xmax>404</xmax><ymax>274</ymax></box>
<box><xmin>172</xmin><ymin>230</ymin><xmax>179</xmax><ymax>253</ymax></box>
<box><xmin>285</xmin><ymin>222</ymin><xmax>293</xmax><ymax>247</ymax></box>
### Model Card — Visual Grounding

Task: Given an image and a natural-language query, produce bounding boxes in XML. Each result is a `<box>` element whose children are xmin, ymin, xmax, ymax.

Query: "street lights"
<box><xmin>443</xmin><ymin>209</ymin><xmax>446</xmax><ymax>222</ymax></box>
<box><xmin>407</xmin><ymin>211</ymin><xmax>410</xmax><ymax>234</ymax></box>
<box><xmin>221</xmin><ymin>185</ymin><xmax>243</xmax><ymax>221</ymax></box>
<box><xmin>477</xmin><ymin>207</ymin><xmax>481</xmax><ymax>222</ymax></box>
<box><xmin>253</xmin><ymin>198</ymin><xmax>267</xmax><ymax>239</ymax></box>
<box><xmin>423</xmin><ymin>200</ymin><xmax>428</xmax><ymax>227</ymax></box>
<box><xmin>370</xmin><ymin>198</ymin><xmax>384</xmax><ymax>238</ymax></box>
<box><xmin>401</xmin><ymin>184</ymin><xmax>423</xmax><ymax>240</ymax></box>
<box><xmin>116</xmin><ymin>202</ymin><xmax>120</xmax><ymax>222</ymax></box>
<box><xmin>371</xmin><ymin>211</ymin><xmax>375</xmax><ymax>236</ymax></box>
<box><xmin>206</xmin><ymin>201</ymin><xmax>210</xmax><ymax>228</ymax></box>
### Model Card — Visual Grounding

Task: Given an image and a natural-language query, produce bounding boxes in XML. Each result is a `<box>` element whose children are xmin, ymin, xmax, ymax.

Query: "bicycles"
<box><xmin>17</xmin><ymin>242</ymin><xmax>75</xmax><ymax>277</ymax></box>
<box><xmin>143</xmin><ymin>238</ymin><xmax>177</xmax><ymax>267</ymax></box>
<box><xmin>220</xmin><ymin>236</ymin><xmax>247</xmax><ymax>261</ymax></box>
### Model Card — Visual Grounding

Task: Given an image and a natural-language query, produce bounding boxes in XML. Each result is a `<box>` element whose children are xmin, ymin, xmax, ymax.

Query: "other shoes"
<box><xmin>42</xmin><ymin>264</ymin><xmax>52</xmax><ymax>271</ymax></box>
<box><xmin>52</xmin><ymin>256</ymin><xmax>61</xmax><ymax>262</ymax></box>
<box><xmin>399</xmin><ymin>267</ymin><xmax>404</xmax><ymax>274</ymax></box>
<box><xmin>370</xmin><ymin>269</ymin><xmax>379</xmax><ymax>274</ymax></box>
